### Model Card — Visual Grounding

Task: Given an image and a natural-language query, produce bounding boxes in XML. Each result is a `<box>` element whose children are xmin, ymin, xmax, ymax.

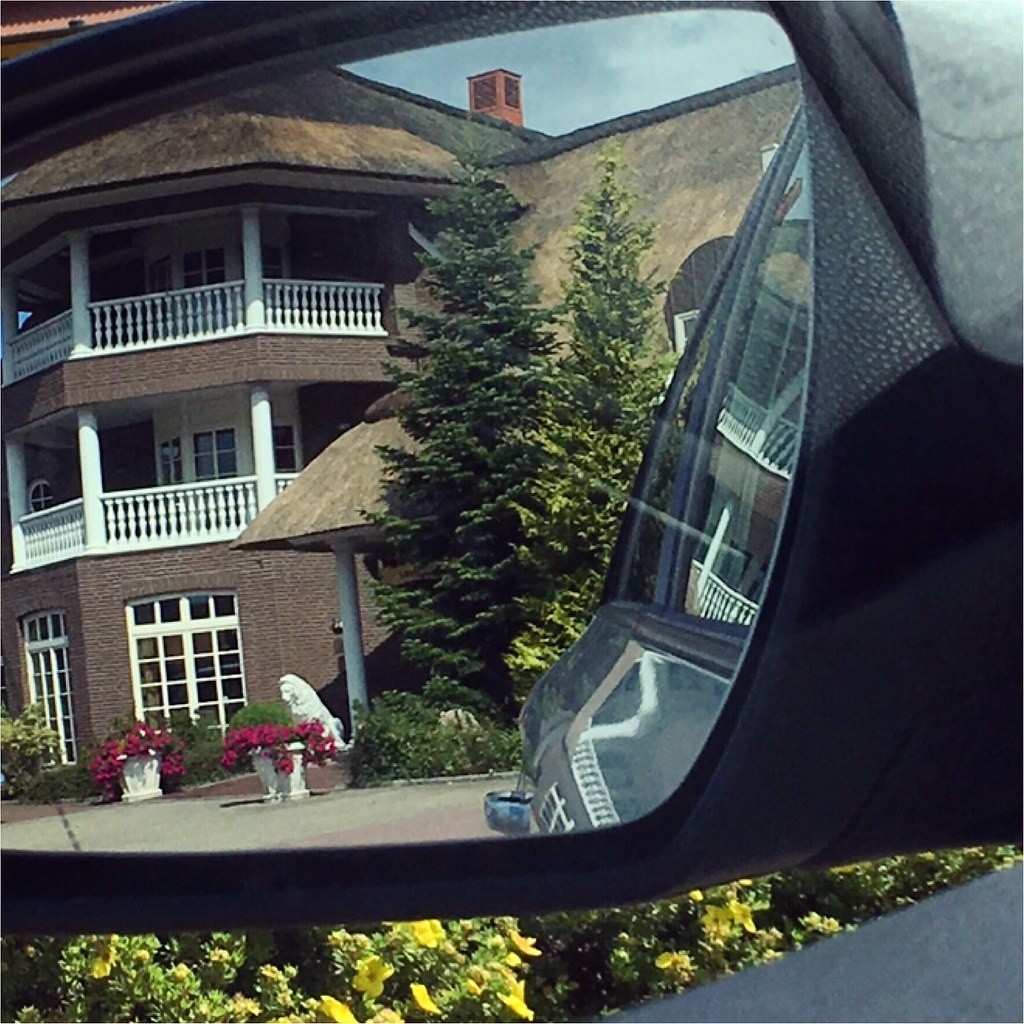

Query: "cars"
<box><xmin>477</xmin><ymin>91</ymin><xmax>812</xmax><ymax>839</ymax></box>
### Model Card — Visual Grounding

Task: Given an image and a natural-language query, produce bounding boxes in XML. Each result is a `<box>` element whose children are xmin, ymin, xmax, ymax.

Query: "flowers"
<box><xmin>223</xmin><ymin>718</ymin><xmax>337</xmax><ymax>775</ymax></box>
<box><xmin>87</xmin><ymin>720</ymin><xmax>183</xmax><ymax>806</ymax></box>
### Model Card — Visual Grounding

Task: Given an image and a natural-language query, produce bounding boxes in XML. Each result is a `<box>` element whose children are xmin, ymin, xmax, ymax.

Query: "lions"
<box><xmin>277</xmin><ymin>674</ymin><xmax>347</xmax><ymax>750</ymax></box>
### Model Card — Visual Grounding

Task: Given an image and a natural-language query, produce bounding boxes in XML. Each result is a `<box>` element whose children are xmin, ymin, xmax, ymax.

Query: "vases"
<box><xmin>249</xmin><ymin>742</ymin><xmax>308</xmax><ymax>806</ymax></box>
<box><xmin>121</xmin><ymin>753</ymin><xmax>162</xmax><ymax>805</ymax></box>
